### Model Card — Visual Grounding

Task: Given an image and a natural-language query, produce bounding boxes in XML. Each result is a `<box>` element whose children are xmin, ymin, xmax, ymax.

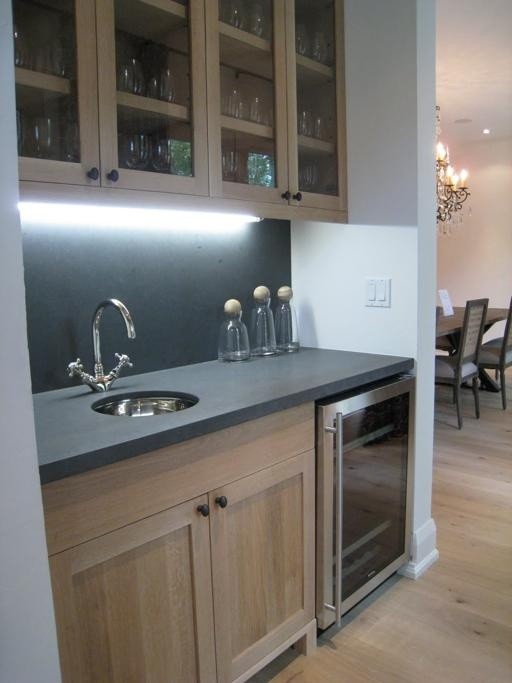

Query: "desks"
<box><xmin>436</xmin><ymin>307</ymin><xmax>509</xmax><ymax>393</ymax></box>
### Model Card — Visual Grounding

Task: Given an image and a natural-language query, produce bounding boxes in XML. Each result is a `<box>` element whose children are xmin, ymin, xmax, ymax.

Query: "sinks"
<box><xmin>90</xmin><ymin>391</ymin><xmax>200</xmax><ymax>417</ymax></box>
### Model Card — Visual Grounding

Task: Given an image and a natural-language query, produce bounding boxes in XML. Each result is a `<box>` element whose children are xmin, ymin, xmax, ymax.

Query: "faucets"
<box><xmin>92</xmin><ymin>298</ymin><xmax>135</xmax><ymax>364</ymax></box>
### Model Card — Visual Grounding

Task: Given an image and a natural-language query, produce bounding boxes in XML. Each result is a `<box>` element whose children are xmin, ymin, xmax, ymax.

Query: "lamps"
<box><xmin>435</xmin><ymin>105</ymin><xmax>471</xmax><ymax>223</ymax></box>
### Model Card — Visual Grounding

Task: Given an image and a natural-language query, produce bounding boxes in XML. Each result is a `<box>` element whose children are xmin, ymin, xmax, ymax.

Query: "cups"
<box><xmin>25</xmin><ymin>0</ymin><xmax>329</xmax><ymax>191</ymax></box>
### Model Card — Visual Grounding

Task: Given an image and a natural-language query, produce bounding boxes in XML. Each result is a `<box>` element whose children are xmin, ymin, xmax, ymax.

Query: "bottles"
<box><xmin>218</xmin><ymin>286</ymin><xmax>299</xmax><ymax>362</ymax></box>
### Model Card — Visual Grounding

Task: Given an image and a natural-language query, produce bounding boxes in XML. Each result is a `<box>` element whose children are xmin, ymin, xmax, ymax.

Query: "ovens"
<box><xmin>315</xmin><ymin>375</ymin><xmax>417</xmax><ymax>631</ymax></box>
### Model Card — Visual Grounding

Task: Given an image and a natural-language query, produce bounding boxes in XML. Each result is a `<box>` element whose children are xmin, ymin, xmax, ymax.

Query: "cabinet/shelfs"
<box><xmin>11</xmin><ymin>0</ymin><xmax>348</xmax><ymax>224</ymax></box>
<box><xmin>41</xmin><ymin>401</ymin><xmax>317</xmax><ymax>683</ymax></box>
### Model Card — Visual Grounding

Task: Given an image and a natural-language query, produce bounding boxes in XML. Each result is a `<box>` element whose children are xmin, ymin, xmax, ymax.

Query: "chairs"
<box><xmin>436</xmin><ymin>299</ymin><xmax>489</xmax><ymax>430</ymax></box>
<box><xmin>479</xmin><ymin>296</ymin><xmax>512</xmax><ymax>411</ymax></box>
